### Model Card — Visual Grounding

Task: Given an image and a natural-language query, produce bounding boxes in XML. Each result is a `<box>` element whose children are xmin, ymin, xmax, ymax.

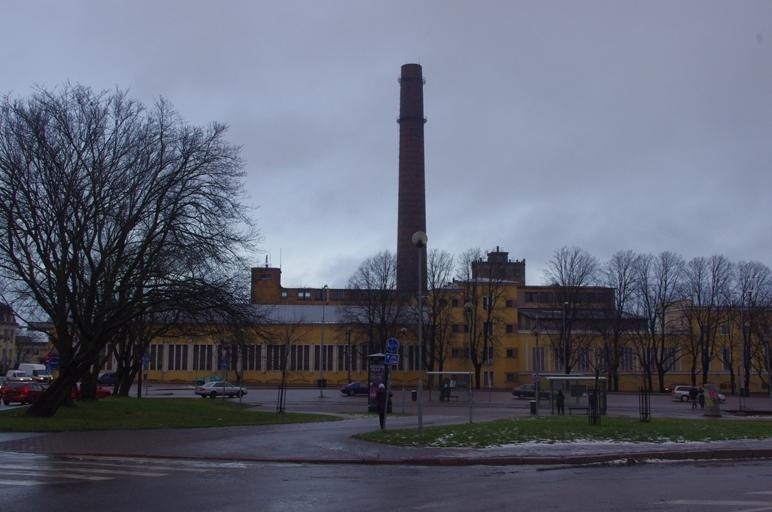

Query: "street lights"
<box><xmin>411</xmin><ymin>230</ymin><xmax>428</xmax><ymax>431</ymax></box>
<box><xmin>562</xmin><ymin>294</ymin><xmax>570</xmax><ymax>375</ymax></box>
<box><xmin>463</xmin><ymin>301</ymin><xmax>474</xmax><ymax>424</ymax></box>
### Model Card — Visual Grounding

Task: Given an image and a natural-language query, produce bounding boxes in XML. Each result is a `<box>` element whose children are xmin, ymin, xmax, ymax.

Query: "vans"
<box><xmin>18</xmin><ymin>363</ymin><xmax>54</xmax><ymax>382</ymax></box>
<box><xmin>671</xmin><ymin>384</ymin><xmax>726</xmax><ymax>403</ymax></box>
<box><xmin>98</xmin><ymin>371</ymin><xmax>118</xmax><ymax>386</ymax></box>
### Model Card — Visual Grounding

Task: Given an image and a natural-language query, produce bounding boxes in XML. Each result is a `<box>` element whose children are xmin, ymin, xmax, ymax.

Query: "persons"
<box><xmin>688</xmin><ymin>386</ymin><xmax>705</xmax><ymax>409</ymax></box>
<box><xmin>374</xmin><ymin>383</ymin><xmax>386</xmax><ymax>430</ymax></box>
<box><xmin>441</xmin><ymin>376</ymin><xmax>451</xmax><ymax>402</ymax></box>
<box><xmin>555</xmin><ymin>390</ymin><xmax>566</xmax><ymax>416</ymax></box>
<box><xmin>347</xmin><ymin>371</ymin><xmax>353</xmax><ymax>384</ymax></box>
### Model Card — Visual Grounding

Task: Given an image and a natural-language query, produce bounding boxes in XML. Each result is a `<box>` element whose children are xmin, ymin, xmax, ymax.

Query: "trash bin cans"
<box><xmin>528</xmin><ymin>402</ymin><xmax>536</xmax><ymax>415</ymax></box>
<box><xmin>411</xmin><ymin>390</ymin><xmax>417</xmax><ymax>401</ymax></box>
<box><xmin>317</xmin><ymin>379</ymin><xmax>327</xmax><ymax>387</ymax></box>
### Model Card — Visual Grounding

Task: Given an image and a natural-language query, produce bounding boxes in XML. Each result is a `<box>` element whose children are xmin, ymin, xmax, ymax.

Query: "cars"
<box><xmin>73</xmin><ymin>381</ymin><xmax>112</xmax><ymax>399</ymax></box>
<box><xmin>195</xmin><ymin>381</ymin><xmax>248</xmax><ymax>399</ymax></box>
<box><xmin>512</xmin><ymin>383</ymin><xmax>559</xmax><ymax>400</ymax></box>
<box><xmin>2</xmin><ymin>381</ymin><xmax>48</xmax><ymax>405</ymax></box>
<box><xmin>340</xmin><ymin>381</ymin><xmax>393</xmax><ymax>397</ymax></box>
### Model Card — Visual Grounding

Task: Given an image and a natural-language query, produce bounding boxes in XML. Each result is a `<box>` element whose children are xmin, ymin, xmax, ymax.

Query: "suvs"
<box><xmin>6</xmin><ymin>369</ymin><xmax>33</xmax><ymax>382</ymax></box>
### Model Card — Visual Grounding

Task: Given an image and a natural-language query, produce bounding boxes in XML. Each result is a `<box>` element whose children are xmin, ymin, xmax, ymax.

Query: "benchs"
<box><xmin>443</xmin><ymin>395</ymin><xmax>460</xmax><ymax>401</ymax></box>
<box><xmin>568</xmin><ymin>408</ymin><xmax>589</xmax><ymax>415</ymax></box>
